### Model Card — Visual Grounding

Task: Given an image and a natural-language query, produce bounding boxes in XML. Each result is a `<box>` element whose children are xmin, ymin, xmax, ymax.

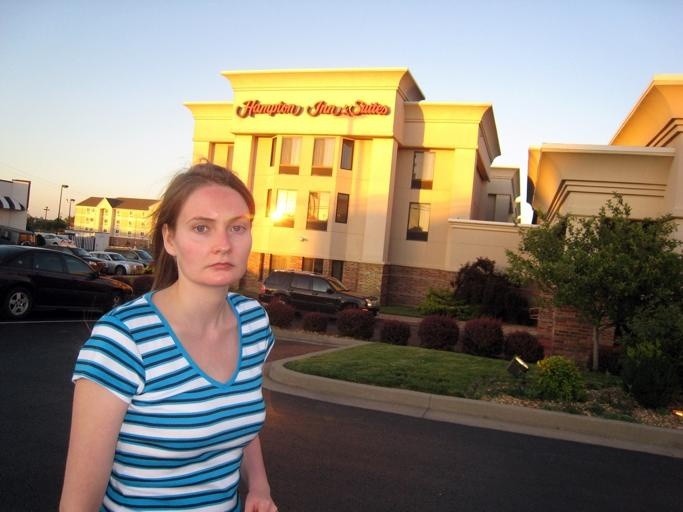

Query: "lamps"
<box><xmin>506</xmin><ymin>355</ymin><xmax>529</xmax><ymax>386</ymax></box>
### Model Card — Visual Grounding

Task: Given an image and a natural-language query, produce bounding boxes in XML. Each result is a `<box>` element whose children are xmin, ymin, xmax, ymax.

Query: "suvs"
<box><xmin>257</xmin><ymin>268</ymin><xmax>381</xmax><ymax>316</ymax></box>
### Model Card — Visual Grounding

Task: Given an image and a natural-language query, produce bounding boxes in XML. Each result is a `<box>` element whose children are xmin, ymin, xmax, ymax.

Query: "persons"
<box><xmin>56</xmin><ymin>163</ymin><xmax>286</xmax><ymax>512</ymax></box>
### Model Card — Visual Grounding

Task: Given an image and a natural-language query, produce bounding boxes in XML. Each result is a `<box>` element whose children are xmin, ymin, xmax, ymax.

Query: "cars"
<box><xmin>1</xmin><ymin>244</ymin><xmax>135</xmax><ymax>322</ymax></box>
<box><xmin>2</xmin><ymin>224</ymin><xmax>154</xmax><ymax>275</ymax></box>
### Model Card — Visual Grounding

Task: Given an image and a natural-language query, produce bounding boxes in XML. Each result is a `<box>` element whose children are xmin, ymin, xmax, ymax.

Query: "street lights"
<box><xmin>68</xmin><ymin>199</ymin><xmax>76</xmax><ymax>223</ymax></box>
<box><xmin>58</xmin><ymin>184</ymin><xmax>69</xmax><ymax>221</ymax></box>
